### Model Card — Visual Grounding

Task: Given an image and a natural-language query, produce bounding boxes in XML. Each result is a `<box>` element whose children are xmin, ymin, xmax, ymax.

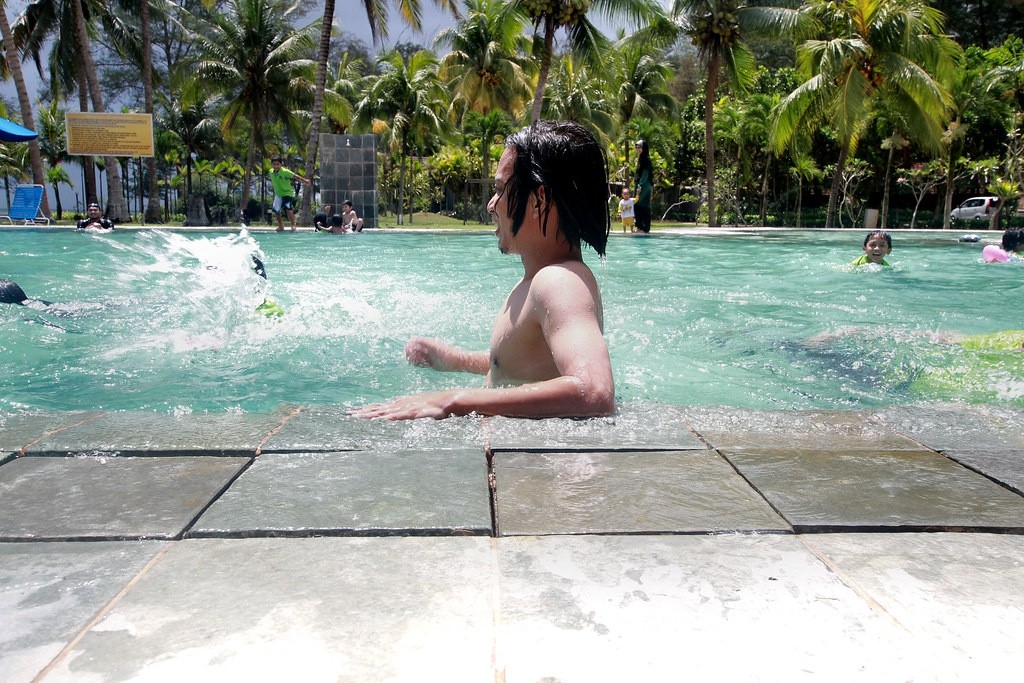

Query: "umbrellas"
<box><xmin>0</xmin><ymin>117</ymin><xmax>38</xmax><ymax>142</ymax></box>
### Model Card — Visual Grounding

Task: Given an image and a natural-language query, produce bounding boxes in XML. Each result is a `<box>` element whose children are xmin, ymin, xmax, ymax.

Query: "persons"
<box><xmin>1001</xmin><ymin>227</ymin><xmax>1024</xmax><ymax>252</ymax></box>
<box><xmin>251</xmin><ymin>254</ymin><xmax>285</xmax><ymax>318</ymax></box>
<box><xmin>361</xmin><ymin>120</ymin><xmax>615</xmax><ymax>420</ymax></box>
<box><xmin>633</xmin><ymin>140</ymin><xmax>653</xmax><ymax>233</ymax></box>
<box><xmin>76</xmin><ymin>203</ymin><xmax>114</xmax><ymax>230</ymax></box>
<box><xmin>340</xmin><ymin>200</ymin><xmax>363</xmax><ymax>232</ymax></box>
<box><xmin>317</xmin><ymin>214</ymin><xmax>345</xmax><ymax>234</ymax></box>
<box><xmin>616</xmin><ymin>188</ymin><xmax>639</xmax><ymax>233</ymax></box>
<box><xmin>0</xmin><ymin>278</ymin><xmax>26</xmax><ymax>304</ymax></box>
<box><xmin>268</xmin><ymin>155</ymin><xmax>310</xmax><ymax>231</ymax></box>
<box><xmin>315</xmin><ymin>205</ymin><xmax>333</xmax><ymax>232</ymax></box>
<box><xmin>850</xmin><ymin>230</ymin><xmax>892</xmax><ymax>267</ymax></box>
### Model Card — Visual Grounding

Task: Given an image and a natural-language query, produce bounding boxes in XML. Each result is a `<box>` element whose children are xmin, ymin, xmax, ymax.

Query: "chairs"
<box><xmin>0</xmin><ymin>183</ymin><xmax>50</xmax><ymax>226</ymax></box>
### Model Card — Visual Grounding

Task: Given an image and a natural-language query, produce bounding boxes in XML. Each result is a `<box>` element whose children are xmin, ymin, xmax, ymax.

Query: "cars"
<box><xmin>950</xmin><ymin>196</ymin><xmax>1002</xmax><ymax>223</ymax></box>
